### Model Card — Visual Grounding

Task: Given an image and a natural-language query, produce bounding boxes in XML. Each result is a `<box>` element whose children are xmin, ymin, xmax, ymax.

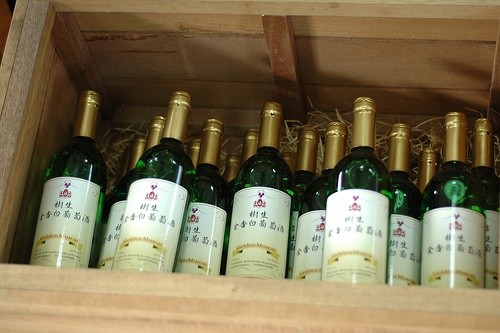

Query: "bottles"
<box><xmin>25</xmin><ymin>90</ymin><xmax>104</xmax><ymax>267</ymax></box>
<box><xmin>96</xmin><ymin>90</ymin><xmax>500</xmax><ymax>290</ymax></box>
